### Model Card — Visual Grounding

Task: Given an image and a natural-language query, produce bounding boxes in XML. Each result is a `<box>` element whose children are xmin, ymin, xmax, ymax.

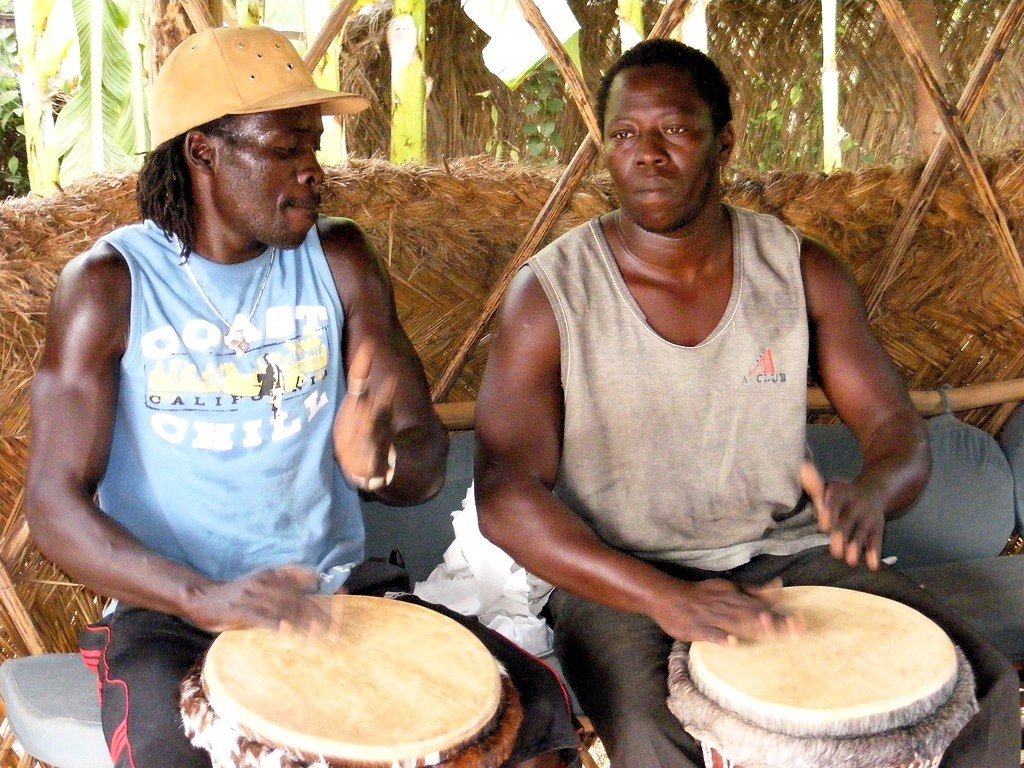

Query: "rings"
<box><xmin>346</xmin><ymin>386</ymin><xmax>369</xmax><ymax>395</ymax></box>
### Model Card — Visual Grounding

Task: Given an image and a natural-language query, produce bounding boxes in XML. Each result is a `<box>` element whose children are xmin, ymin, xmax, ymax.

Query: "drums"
<box><xmin>175</xmin><ymin>590</ymin><xmax>523</xmax><ymax>768</ymax></box>
<box><xmin>665</xmin><ymin>582</ymin><xmax>979</xmax><ymax>768</ymax></box>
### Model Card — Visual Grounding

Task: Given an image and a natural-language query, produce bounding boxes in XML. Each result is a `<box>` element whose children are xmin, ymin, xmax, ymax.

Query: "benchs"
<box><xmin>0</xmin><ymin>376</ymin><xmax>1024</xmax><ymax>768</ymax></box>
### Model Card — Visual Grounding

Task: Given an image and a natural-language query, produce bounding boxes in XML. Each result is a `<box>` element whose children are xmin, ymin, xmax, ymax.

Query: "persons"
<box><xmin>25</xmin><ymin>26</ymin><xmax>586</xmax><ymax>768</ymax></box>
<box><xmin>472</xmin><ymin>37</ymin><xmax>1021</xmax><ymax>768</ymax></box>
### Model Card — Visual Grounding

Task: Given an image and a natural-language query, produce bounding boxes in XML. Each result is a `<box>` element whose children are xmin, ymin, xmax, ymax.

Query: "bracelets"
<box><xmin>349</xmin><ymin>444</ymin><xmax>397</xmax><ymax>491</ymax></box>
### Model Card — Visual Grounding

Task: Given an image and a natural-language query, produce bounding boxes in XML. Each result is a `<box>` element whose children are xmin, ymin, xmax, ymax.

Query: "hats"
<box><xmin>147</xmin><ymin>25</ymin><xmax>370</xmax><ymax>151</ymax></box>
